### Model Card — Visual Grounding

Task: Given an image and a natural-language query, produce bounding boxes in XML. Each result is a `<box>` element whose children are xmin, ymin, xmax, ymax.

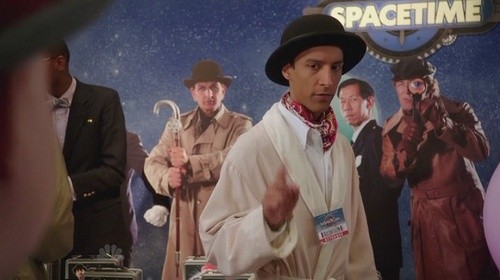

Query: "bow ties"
<box><xmin>49</xmin><ymin>98</ymin><xmax>70</xmax><ymax>111</ymax></box>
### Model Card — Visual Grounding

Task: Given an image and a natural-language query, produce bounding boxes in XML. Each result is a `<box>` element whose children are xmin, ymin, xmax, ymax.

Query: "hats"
<box><xmin>184</xmin><ymin>60</ymin><xmax>232</xmax><ymax>90</ymax></box>
<box><xmin>392</xmin><ymin>59</ymin><xmax>434</xmax><ymax>80</ymax></box>
<box><xmin>264</xmin><ymin>13</ymin><xmax>368</xmax><ymax>87</ymax></box>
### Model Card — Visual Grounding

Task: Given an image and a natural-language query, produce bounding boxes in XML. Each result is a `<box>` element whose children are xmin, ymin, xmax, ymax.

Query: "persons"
<box><xmin>71</xmin><ymin>263</ymin><xmax>87</xmax><ymax>280</ymax></box>
<box><xmin>0</xmin><ymin>0</ymin><xmax>104</xmax><ymax>280</ymax></box>
<box><xmin>379</xmin><ymin>57</ymin><xmax>500</xmax><ymax>280</ymax></box>
<box><xmin>144</xmin><ymin>60</ymin><xmax>253</xmax><ymax>280</ymax></box>
<box><xmin>335</xmin><ymin>78</ymin><xmax>405</xmax><ymax>280</ymax></box>
<box><xmin>40</xmin><ymin>37</ymin><xmax>133</xmax><ymax>280</ymax></box>
<box><xmin>200</xmin><ymin>14</ymin><xmax>376</xmax><ymax>280</ymax></box>
